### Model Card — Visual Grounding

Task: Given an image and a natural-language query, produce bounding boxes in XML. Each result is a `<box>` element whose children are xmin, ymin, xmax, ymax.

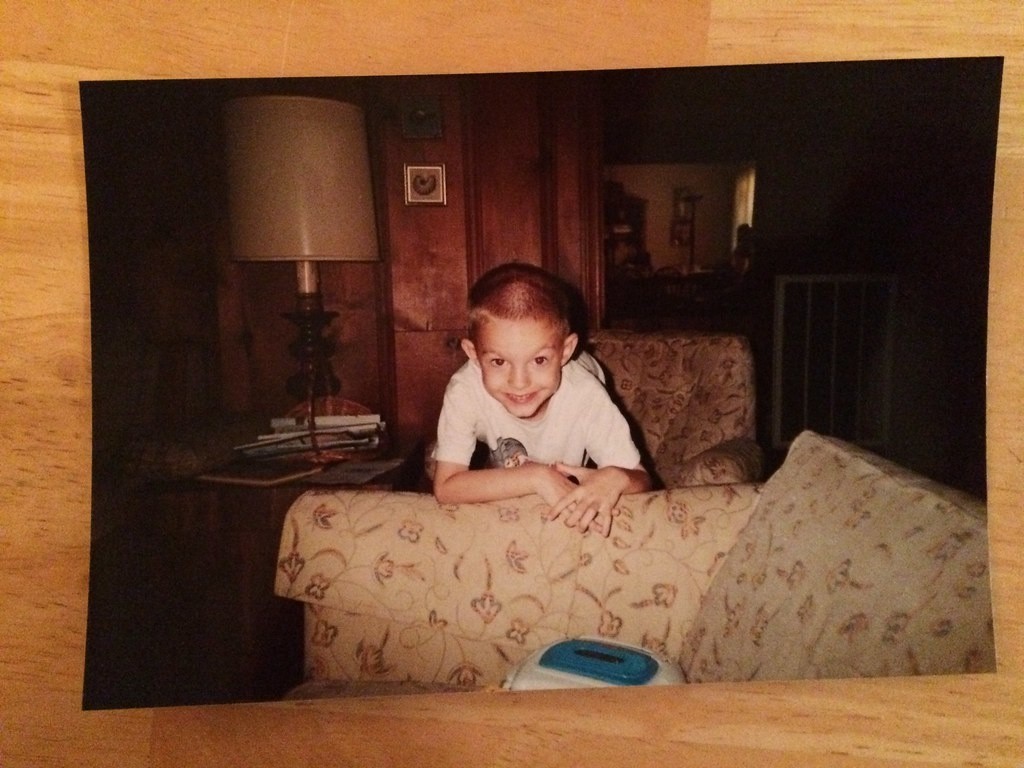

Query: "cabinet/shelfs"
<box><xmin>114</xmin><ymin>423</ymin><xmax>414</xmax><ymax>701</ymax></box>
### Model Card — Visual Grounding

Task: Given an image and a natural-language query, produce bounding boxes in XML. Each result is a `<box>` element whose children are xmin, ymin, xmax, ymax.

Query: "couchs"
<box><xmin>425</xmin><ymin>332</ymin><xmax>758</xmax><ymax>488</ymax></box>
<box><xmin>274</xmin><ymin>430</ymin><xmax>999</xmax><ymax>702</ymax></box>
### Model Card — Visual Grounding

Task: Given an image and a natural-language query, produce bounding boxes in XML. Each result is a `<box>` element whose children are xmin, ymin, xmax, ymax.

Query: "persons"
<box><xmin>430</xmin><ymin>260</ymin><xmax>652</xmax><ymax>539</ymax></box>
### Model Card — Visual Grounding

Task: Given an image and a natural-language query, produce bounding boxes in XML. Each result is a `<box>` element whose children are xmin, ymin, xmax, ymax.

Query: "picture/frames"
<box><xmin>398</xmin><ymin>88</ymin><xmax>445</xmax><ymax>139</ymax></box>
<box><xmin>403</xmin><ymin>162</ymin><xmax>448</xmax><ymax>207</ymax></box>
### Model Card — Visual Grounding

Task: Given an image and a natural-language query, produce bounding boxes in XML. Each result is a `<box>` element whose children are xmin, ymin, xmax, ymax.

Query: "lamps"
<box><xmin>216</xmin><ymin>96</ymin><xmax>381</xmax><ymax>459</ymax></box>
